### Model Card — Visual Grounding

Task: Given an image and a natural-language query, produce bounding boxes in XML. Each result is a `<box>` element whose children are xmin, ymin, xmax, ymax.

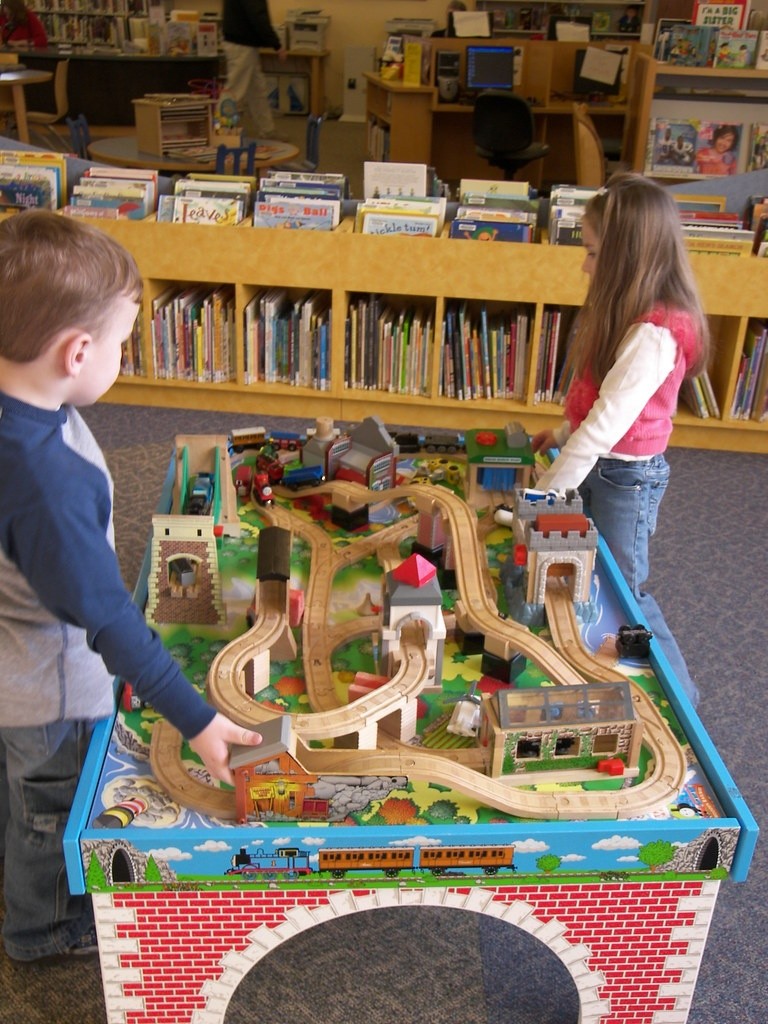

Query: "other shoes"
<box><xmin>259</xmin><ymin>130</ymin><xmax>288</xmax><ymax>144</ymax></box>
<box><xmin>63</xmin><ymin>919</ymin><xmax>99</xmax><ymax>955</ymax></box>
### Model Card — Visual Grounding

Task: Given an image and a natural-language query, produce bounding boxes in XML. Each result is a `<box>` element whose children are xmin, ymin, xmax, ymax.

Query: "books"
<box><xmin>22</xmin><ymin>0</ymin><xmax>199</xmax><ymax>57</ymax></box>
<box><xmin>663</xmin><ymin>194</ymin><xmax>768</xmax><ymax>257</ymax></box>
<box><xmin>591</xmin><ymin>9</ymin><xmax>612</xmax><ymax>31</ymax></box>
<box><xmin>677</xmin><ymin>327</ymin><xmax>768</xmax><ymax>423</ymax></box>
<box><xmin>118</xmin><ymin>285</ymin><xmax>576</xmax><ymax>407</ymax></box>
<box><xmin>0</xmin><ymin>150</ymin><xmax>600</xmax><ymax>247</ymax></box>
<box><xmin>643</xmin><ymin>26</ymin><xmax>768</xmax><ymax>176</ymax></box>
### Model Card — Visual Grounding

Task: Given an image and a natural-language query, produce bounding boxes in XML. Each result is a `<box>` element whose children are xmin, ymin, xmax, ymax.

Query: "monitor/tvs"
<box><xmin>546</xmin><ymin>14</ymin><xmax>593</xmax><ymax>41</ymax></box>
<box><xmin>573</xmin><ymin>49</ymin><xmax>623</xmax><ymax>102</ymax></box>
<box><xmin>445</xmin><ymin>11</ymin><xmax>494</xmax><ymax>39</ymax></box>
<box><xmin>465</xmin><ymin>45</ymin><xmax>515</xmax><ymax>90</ymax></box>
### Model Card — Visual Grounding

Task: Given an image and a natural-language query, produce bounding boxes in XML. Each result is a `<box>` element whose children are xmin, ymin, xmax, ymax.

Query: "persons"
<box><xmin>213</xmin><ymin>0</ymin><xmax>288</xmax><ymax>145</ymax></box>
<box><xmin>696</xmin><ymin>125</ymin><xmax>740</xmax><ymax>176</ymax></box>
<box><xmin>617</xmin><ymin>5</ymin><xmax>641</xmax><ymax>32</ymax></box>
<box><xmin>430</xmin><ymin>0</ymin><xmax>467</xmax><ymax>38</ymax></box>
<box><xmin>655</xmin><ymin>129</ymin><xmax>673</xmax><ymax>164</ymax></box>
<box><xmin>671</xmin><ymin>136</ymin><xmax>696</xmax><ymax>166</ymax></box>
<box><xmin>531</xmin><ymin>170</ymin><xmax>708</xmax><ymax>712</ymax></box>
<box><xmin>0</xmin><ymin>0</ymin><xmax>48</xmax><ymax>49</ymax></box>
<box><xmin>1</xmin><ymin>211</ymin><xmax>263</xmax><ymax>962</ymax></box>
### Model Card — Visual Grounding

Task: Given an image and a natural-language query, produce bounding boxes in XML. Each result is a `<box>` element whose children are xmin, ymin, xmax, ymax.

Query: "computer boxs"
<box><xmin>435</xmin><ymin>48</ymin><xmax>462</xmax><ymax>103</ymax></box>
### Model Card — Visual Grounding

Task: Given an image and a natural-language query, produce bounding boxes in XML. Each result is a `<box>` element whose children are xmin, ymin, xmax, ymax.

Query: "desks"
<box><xmin>1</xmin><ymin>69</ymin><xmax>53</xmax><ymax>147</ymax></box>
<box><xmin>0</xmin><ymin>49</ymin><xmax>329</xmax><ymax>126</ymax></box>
<box><xmin>88</xmin><ymin>136</ymin><xmax>301</xmax><ymax>177</ymax></box>
<box><xmin>61</xmin><ymin>436</ymin><xmax>758</xmax><ymax>1023</ymax></box>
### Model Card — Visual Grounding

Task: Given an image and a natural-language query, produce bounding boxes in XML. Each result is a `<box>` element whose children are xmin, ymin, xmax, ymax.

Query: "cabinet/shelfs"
<box><xmin>622</xmin><ymin>43</ymin><xmax>768</xmax><ymax>214</ymax></box>
<box><xmin>0</xmin><ymin>179</ymin><xmax>768</xmax><ymax>455</ymax></box>
<box><xmin>361</xmin><ymin>32</ymin><xmax>657</xmax><ymax>180</ymax></box>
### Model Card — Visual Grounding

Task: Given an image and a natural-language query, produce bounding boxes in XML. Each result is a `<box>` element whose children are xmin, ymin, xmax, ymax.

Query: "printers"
<box><xmin>385</xmin><ymin>16</ymin><xmax>438</xmax><ymax>41</ymax></box>
<box><xmin>284</xmin><ymin>14</ymin><xmax>331</xmax><ymax>53</ymax></box>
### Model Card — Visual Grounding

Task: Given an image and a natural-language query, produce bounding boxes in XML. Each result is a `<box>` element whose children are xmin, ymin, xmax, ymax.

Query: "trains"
<box><xmin>387</xmin><ymin>430</ymin><xmax>460</xmax><ymax>455</ymax></box>
<box><xmin>228</xmin><ymin>425</ymin><xmax>325</xmax><ymax>507</ymax></box>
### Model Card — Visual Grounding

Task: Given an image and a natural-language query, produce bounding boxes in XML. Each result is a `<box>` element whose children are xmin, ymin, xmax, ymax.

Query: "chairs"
<box><xmin>470</xmin><ymin>89</ymin><xmax>550</xmax><ymax>181</ymax></box>
<box><xmin>269</xmin><ymin>113</ymin><xmax>322</xmax><ymax>173</ymax></box>
<box><xmin>215</xmin><ymin>143</ymin><xmax>256</xmax><ymax>178</ymax></box>
<box><xmin>8</xmin><ymin>58</ymin><xmax>76</xmax><ymax>160</ymax></box>
